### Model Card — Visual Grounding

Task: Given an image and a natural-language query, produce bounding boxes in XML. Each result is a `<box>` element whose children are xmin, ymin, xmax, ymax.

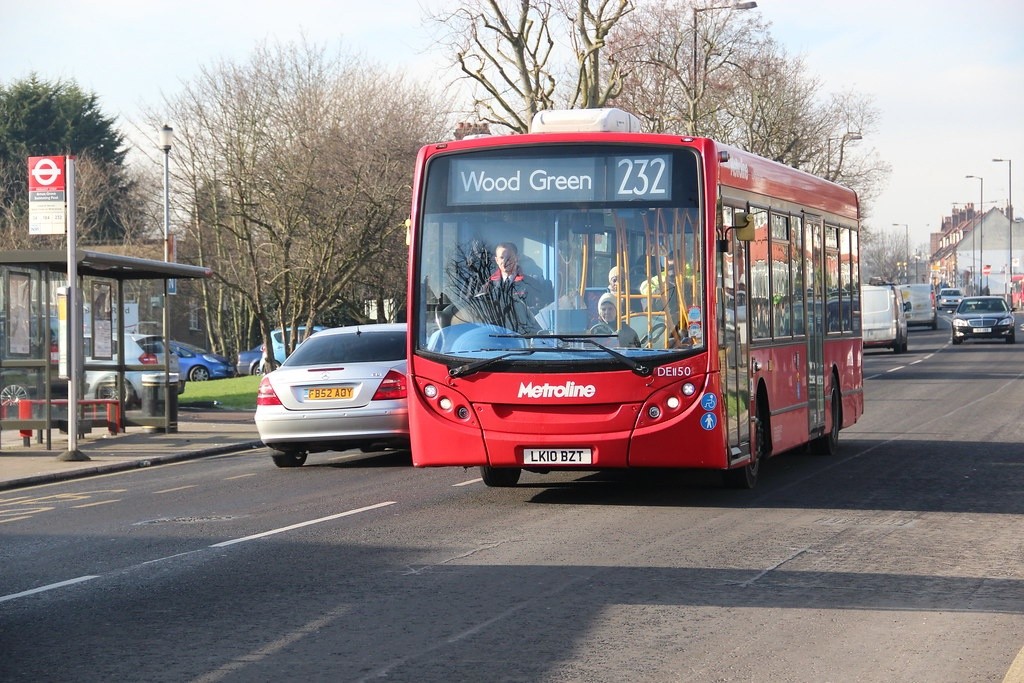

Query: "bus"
<box><xmin>407</xmin><ymin>107</ymin><xmax>866</xmax><ymax>495</ymax></box>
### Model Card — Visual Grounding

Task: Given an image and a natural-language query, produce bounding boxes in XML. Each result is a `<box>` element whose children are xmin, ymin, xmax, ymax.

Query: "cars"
<box><xmin>0</xmin><ymin>300</ymin><xmax>69</xmax><ymax>419</ymax></box>
<box><xmin>255</xmin><ymin>324</ymin><xmax>412</xmax><ymax>472</ymax></box>
<box><xmin>721</xmin><ymin>282</ymin><xmax>861</xmax><ymax>334</ymax></box>
<box><xmin>948</xmin><ymin>295</ymin><xmax>1018</xmax><ymax>346</ymax></box>
<box><xmin>260</xmin><ymin>326</ymin><xmax>330</xmax><ymax>375</ymax></box>
<box><xmin>163</xmin><ymin>340</ymin><xmax>235</xmax><ymax>383</ymax></box>
<box><xmin>79</xmin><ymin>331</ymin><xmax>179</xmax><ymax>411</ymax></box>
<box><xmin>937</xmin><ymin>288</ymin><xmax>964</xmax><ymax>312</ymax></box>
<box><xmin>237</xmin><ymin>341</ymin><xmax>263</xmax><ymax>376</ymax></box>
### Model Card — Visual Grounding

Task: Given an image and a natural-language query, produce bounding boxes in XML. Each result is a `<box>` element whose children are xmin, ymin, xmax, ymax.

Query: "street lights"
<box><xmin>990</xmin><ymin>158</ymin><xmax>1013</xmax><ymax>307</ymax></box>
<box><xmin>950</xmin><ymin>201</ymin><xmax>975</xmax><ymax>295</ymax></box>
<box><xmin>963</xmin><ymin>174</ymin><xmax>984</xmax><ymax>295</ymax></box>
<box><xmin>826</xmin><ymin>136</ymin><xmax>862</xmax><ymax>180</ymax></box>
<box><xmin>160</xmin><ymin>122</ymin><xmax>173</xmax><ymax>437</ymax></box>
<box><xmin>892</xmin><ymin>221</ymin><xmax>910</xmax><ymax>282</ymax></box>
<box><xmin>692</xmin><ymin>1</ymin><xmax>758</xmax><ymax>139</ymax></box>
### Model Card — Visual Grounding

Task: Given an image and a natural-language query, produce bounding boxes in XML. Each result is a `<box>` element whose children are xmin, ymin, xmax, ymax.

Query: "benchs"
<box><xmin>18</xmin><ymin>399</ymin><xmax>121</xmax><ymax>447</ymax></box>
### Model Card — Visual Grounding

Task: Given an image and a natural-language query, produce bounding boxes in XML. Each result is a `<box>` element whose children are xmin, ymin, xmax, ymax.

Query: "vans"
<box><xmin>855</xmin><ymin>284</ymin><xmax>909</xmax><ymax>354</ymax></box>
<box><xmin>1012</xmin><ymin>275</ymin><xmax>1024</xmax><ymax>311</ymax></box>
<box><xmin>898</xmin><ymin>283</ymin><xmax>939</xmax><ymax>330</ymax></box>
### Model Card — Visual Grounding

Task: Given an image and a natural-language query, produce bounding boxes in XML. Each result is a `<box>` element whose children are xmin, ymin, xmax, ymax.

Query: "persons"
<box><xmin>476</xmin><ymin>241</ymin><xmax>551</xmax><ymax>337</ymax></box>
<box><xmin>583</xmin><ymin>249</ymin><xmax>693</xmax><ymax>349</ymax></box>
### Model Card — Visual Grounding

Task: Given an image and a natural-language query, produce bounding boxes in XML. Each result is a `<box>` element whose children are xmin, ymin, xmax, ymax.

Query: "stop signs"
<box><xmin>983</xmin><ymin>267</ymin><xmax>989</xmax><ymax>274</ymax></box>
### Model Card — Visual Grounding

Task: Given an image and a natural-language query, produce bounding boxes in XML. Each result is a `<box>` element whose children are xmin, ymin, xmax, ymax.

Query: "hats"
<box><xmin>598</xmin><ymin>293</ymin><xmax>617</xmax><ymax>315</ymax></box>
<box><xmin>608</xmin><ymin>266</ymin><xmax>625</xmax><ymax>281</ymax></box>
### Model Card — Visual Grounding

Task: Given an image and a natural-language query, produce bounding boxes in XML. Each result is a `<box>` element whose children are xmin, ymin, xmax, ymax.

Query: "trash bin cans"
<box><xmin>141</xmin><ymin>372</ymin><xmax>179</xmax><ymax>435</ymax></box>
